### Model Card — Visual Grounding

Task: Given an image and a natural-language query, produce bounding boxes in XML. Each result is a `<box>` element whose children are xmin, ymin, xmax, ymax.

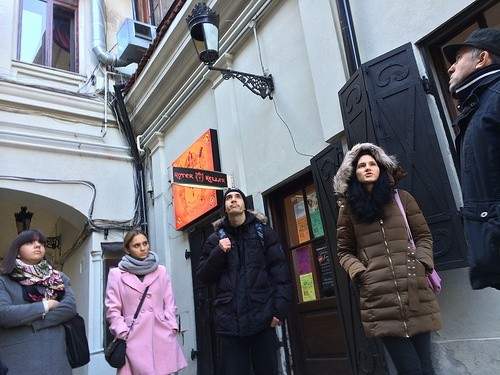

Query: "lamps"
<box><xmin>13</xmin><ymin>206</ymin><xmax>61</xmax><ymax>252</ymax></box>
<box><xmin>184</xmin><ymin>2</ymin><xmax>274</xmax><ymax>100</ymax></box>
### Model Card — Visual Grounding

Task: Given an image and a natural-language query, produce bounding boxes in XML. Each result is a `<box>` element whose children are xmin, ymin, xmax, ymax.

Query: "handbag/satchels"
<box><xmin>104</xmin><ymin>338</ymin><xmax>127</xmax><ymax>369</ymax></box>
<box><xmin>427</xmin><ymin>269</ymin><xmax>442</xmax><ymax>294</ymax></box>
<box><xmin>64</xmin><ymin>313</ymin><xmax>91</xmax><ymax>367</ymax></box>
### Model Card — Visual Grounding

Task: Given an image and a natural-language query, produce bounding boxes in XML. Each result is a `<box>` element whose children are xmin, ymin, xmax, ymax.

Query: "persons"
<box><xmin>442</xmin><ymin>28</ymin><xmax>500</xmax><ymax>291</ymax></box>
<box><xmin>333</xmin><ymin>142</ymin><xmax>443</xmax><ymax>375</ymax></box>
<box><xmin>196</xmin><ymin>188</ymin><xmax>293</xmax><ymax>375</ymax></box>
<box><xmin>104</xmin><ymin>229</ymin><xmax>188</xmax><ymax>375</ymax></box>
<box><xmin>0</xmin><ymin>229</ymin><xmax>77</xmax><ymax>375</ymax></box>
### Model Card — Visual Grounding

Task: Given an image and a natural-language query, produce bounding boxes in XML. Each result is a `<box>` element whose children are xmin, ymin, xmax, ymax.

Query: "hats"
<box><xmin>440</xmin><ymin>27</ymin><xmax>500</xmax><ymax>64</ymax></box>
<box><xmin>223</xmin><ymin>186</ymin><xmax>247</xmax><ymax>209</ymax></box>
